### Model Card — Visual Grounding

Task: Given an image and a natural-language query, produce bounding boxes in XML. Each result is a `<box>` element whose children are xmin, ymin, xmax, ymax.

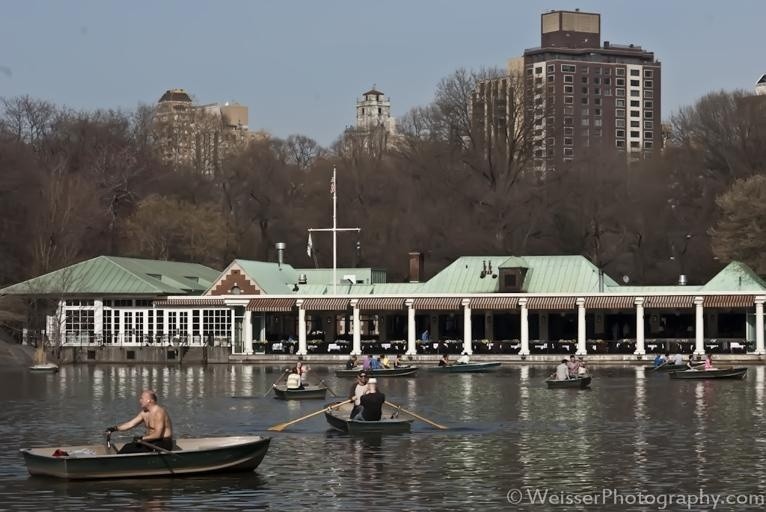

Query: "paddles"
<box><xmin>267</xmin><ymin>399</ymin><xmax>350</xmax><ymax>432</ymax></box>
<box><xmin>383</xmin><ymin>400</ymin><xmax>446</xmax><ymax>429</ymax></box>
<box><xmin>134</xmin><ymin>437</ymin><xmax>181</xmax><ymax>458</ymax></box>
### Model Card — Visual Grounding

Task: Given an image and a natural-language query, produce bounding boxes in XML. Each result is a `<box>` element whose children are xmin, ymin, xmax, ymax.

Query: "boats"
<box><xmin>643</xmin><ymin>361</ymin><xmax>749</xmax><ymax>380</ymax></box>
<box><xmin>273</xmin><ymin>378</ymin><xmax>328</xmax><ymax>400</ymax></box>
<box><xmin>323</xmin><ymin>401</ymin><xmax>414</xmax><ymax>435</ymax></box>
<box><xmin>545</xmin><ymin>371</ymin><xmax>592</xmax><ymax>389</ymax></box>
<box><xmin>27</xmin><ymin>362</ymin><xmax>60</xmax><ymax>375</ymax></box>
<box><xmin>429</xmin><ymin>361</ymin><xmax>502</xmax><ymax>373</ymax></box>
<box><xmin>19</xmin><ymin>434</ymin><xmax>273</xmax><ymax>480</ymax></box>
<box><xmin>336</xmin><ymin>363</ymin><xmax>418</xmax><ymax>378</ymax></box>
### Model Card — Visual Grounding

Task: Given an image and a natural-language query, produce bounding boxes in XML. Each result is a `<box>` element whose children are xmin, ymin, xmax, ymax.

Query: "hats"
<box><xmin>367</xmin><ymin>377</ymin><xmax>378</xmax><ymax>384</ymax></box>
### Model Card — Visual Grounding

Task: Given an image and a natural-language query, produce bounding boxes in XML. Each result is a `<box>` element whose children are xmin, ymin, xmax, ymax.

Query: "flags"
<box><xmin>330</xmin><ymin>176</ymin><xmax>336</xmax><ymax>194</ymax></box>
<box><xmin>306</xmin><ymin>232</ymin><xmax>313</xmax><ymax>257</ymax></box>
<box><xmin>355</xmin><ymin>230</ymin><xmax>362</xmax><ymax>257</ymax></box>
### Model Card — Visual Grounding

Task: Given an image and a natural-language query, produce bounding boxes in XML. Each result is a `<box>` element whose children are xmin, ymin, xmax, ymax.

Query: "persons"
<box><xmin>286</xmin><ymin>366</ymin><xmax>306</xmax><ymax>390</ymax></box>
<box><xmin>352</xmin><ymin>378</ymin><xmax>385</xmax><ymax>421</ymax></box>
<box><xmin>455</xmin><ymin>352</ymin><xmax>469</xmax><ymax>365</ymax></box>
<box><xmin>577</xmin><ymin>356</ymin><xmax>585</xmax><ymax>368</ymax></box>
<box><xmin>345</xmin><ymin>352</ymin><xmax>402</xmax><ymax>369</ymax></box>
<box><xmin>439</xmin><ymin>353</ymin><xmax>448</xmax><ymax>366</ymax></box>
<box><xmin>286</xmin><ymin>361</ymin><xmax>311</xmax><ymax>386</ymax></box>
<box><xmin>422</xmin><ymin>329</ymin><xmax>428</xmax><ymax>340</ymax></box>
<box><xmin>653</xmin><ymin>353</ymin><xmax>718</xmax><ymax>371</ymax></box>
<box><xmin>105</xmin><ymin>390</ymin><xmax>172</xmax><ymax>454</ymax></box>
<box><xmin>555</xmin><ymin>359</ymin><xmax>570</xmax><ymax>380</ymax></box>
<box><xmin>568</xmin><ymin>355</ymin><xmax>580</xmax><ymax>380</ymax></box>
<box><xmin>348</xmin><ymin>370</ymin><xmax>369</xmax><ymax>418</ymax></box>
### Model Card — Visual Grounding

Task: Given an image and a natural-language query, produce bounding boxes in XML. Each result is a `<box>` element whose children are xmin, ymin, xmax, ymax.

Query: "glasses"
<box><xmin>360</xmin><ymin>375</ymin><xmax>367</xmax><ymax>378</ymax></box>
